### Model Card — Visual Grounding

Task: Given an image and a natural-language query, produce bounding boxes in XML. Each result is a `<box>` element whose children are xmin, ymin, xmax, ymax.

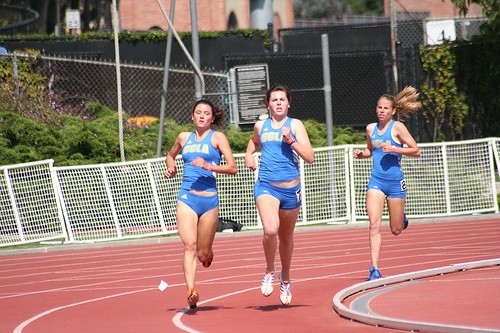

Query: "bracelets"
<box><xmin>392</xmin><ymin>146</ymin><xmax>395</xmax><ymax>152</ymax></box>
<box><xmin>209</xmin><ymin>163</ymin><xmax>213</xmax><ymax>171</ymax></box>
<box><xmin>289</xmin><ymin>140</ymin><xmax>294</xmax><ymax>146</ymax></box>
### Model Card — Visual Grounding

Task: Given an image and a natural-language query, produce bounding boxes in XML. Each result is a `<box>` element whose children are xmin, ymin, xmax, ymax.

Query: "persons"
<box><xmin>245</xmin><ymin>86</ymin><xmax>315</xmax><ymax>305</ymax></box>
<box><xmin>352</xmin><ymin>86</ymin><xmax>422</xmax><ymax>283</ymax></box>
<box><xmin>165</xmin><ymin>99</ymin><xmax>237</xmax><ymax>307</ymax></box>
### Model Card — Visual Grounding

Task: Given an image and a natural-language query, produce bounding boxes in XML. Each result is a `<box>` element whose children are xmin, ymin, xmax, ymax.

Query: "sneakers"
<box><xmin>261</xmin><ymin>267</ymin><xmax>276</xmax><ymax>297</ymax></box>
<box><xmin>278</xmin><ymin>270</ymin><xmax>292</xmax><ymax>305</ymax></box>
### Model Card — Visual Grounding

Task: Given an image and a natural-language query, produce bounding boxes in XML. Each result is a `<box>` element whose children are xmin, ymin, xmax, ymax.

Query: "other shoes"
<box><xmin>367</xmin><ymin>264</ymin><xmax>383</xmax><ymax>281</ymax></box>
<box><xmin>187</xmin><ymin>288</ymin><xmax>200</xmax><ymax>306</ymax></box>
<box><xmin>202</xmin><ymin>251</ymin><xmax>214</xmax><ymax>268</ymax></box>
<box><xmin>403</xmin><ymin>212</ymin><xmax>408</xmax><ymax>230</ymax></box>
